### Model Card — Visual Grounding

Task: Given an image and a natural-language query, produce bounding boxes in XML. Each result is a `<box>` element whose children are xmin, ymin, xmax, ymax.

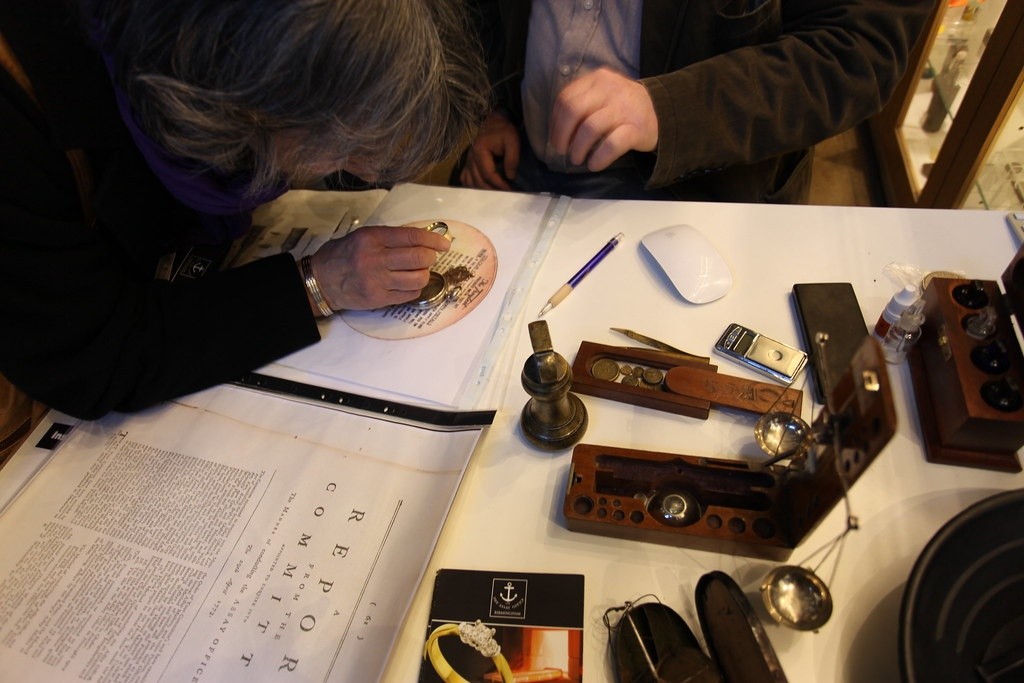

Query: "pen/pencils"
<box><xmin>536</xmin><ymin>231</ymin><xmax>625</xmax><ymax>319</ymax></box>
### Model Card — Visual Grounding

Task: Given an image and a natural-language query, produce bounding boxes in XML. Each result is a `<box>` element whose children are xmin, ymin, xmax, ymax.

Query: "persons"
<box><xmin>0</xmin><ymin>0</ymin><xmax>493</xmax><ymax>422</ymax></box>
<box><xmin>447</xmin><ymin>0</ymin><xmax>942</xmax><ymax>206</ymax></box>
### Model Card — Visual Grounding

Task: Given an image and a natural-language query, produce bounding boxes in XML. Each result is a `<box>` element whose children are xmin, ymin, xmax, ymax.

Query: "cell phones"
<box><xmin>715</xmin><ymin>323</ymin><xmax>809</xmax><ymax>387</ymax></box>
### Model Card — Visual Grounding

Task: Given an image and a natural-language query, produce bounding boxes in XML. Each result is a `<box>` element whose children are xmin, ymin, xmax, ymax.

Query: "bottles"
<box><xmin>872</xmin><ymin>283</ymin><xmax>925</xmax><ymax>365</ymax></box>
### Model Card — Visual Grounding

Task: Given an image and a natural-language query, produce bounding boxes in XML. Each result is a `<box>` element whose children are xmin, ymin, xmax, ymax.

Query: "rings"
<box><xmin>424</xmin><ymin>618</ymin><xmax>517</xmax><ymax>683</ymax></box>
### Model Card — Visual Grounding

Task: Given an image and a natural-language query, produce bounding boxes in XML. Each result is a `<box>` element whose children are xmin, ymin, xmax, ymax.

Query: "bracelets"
<box><xmin>301</xmin><ymin>254</ymin><xmax>335</xmax><ymax>317</ymax></box>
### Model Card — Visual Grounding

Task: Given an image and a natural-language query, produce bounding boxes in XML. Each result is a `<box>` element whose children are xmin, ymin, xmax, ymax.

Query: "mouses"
<box><xmin>641</xmin><ymin>224</ymin><xmax>733</xmax><ymax>303</ymax></box>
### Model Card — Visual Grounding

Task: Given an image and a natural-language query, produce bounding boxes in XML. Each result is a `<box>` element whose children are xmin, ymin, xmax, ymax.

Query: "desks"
<box><xmin>0</xmin><ymin>189</ymin><xmax>1024</xmax><ymax>683</ymax></box>
